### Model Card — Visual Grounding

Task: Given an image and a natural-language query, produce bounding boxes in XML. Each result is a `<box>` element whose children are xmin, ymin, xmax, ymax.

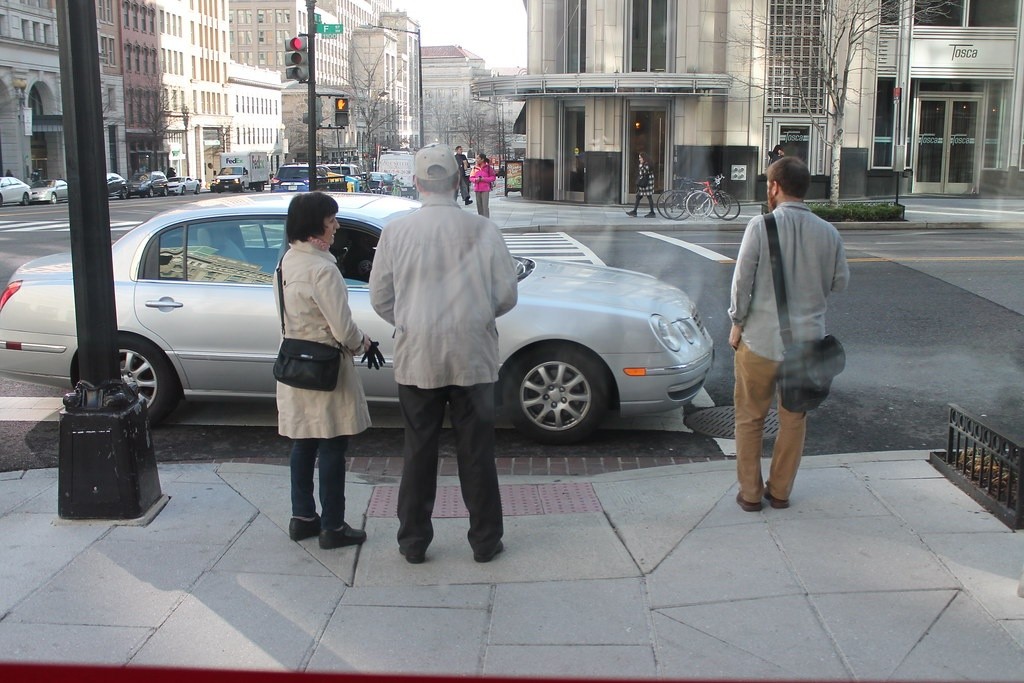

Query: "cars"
<box><xmin>125</xmin><ymin>170</ymin><xmax>170</xmax><ymax>198</ymax></box>
<box><xmin>0</xmin><ymin>176</ymin><xmax>32</xmax><ymax>206</ymax></box>
<box><xmin>29</xmin><ymin>179</ymin><xmax>69</xmax><ymax>204</ymax></box>
<box><xmin>106</xmin><ymin>172</ymin><xmax>128</xmax><ymax>200</ymax></box>
<box><xmin>0</xmin><ymin>191</ymin><xmax>718</xmax><ymax>445</ymax></box>
<box><xmin>166</xmin><ymin>176</ymin><xmax>201</xmax><ymax>196</ymax></box>
<box><xmin>362</xmin><ymin>171</ymin><xmax>388</xmax><ymax>191</ymax></box>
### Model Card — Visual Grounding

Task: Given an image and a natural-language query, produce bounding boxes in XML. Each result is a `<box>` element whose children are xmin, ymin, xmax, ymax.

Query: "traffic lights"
<box><xmin>283</xmin><ymin>37</ymin><xmax>309</xmax><ymax>81</ymax></box>
<box><xmin>334</xmin><ymin>97</ymin><xmax>349</xmax><ymax>126</ymax></box>
<box><xmin>302</xmin><ymin>99</ymin><xmax>310</xmax><ymax>123</ymax></box>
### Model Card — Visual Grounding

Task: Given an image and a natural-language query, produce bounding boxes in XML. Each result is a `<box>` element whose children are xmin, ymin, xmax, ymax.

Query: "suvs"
<box><xmin>270</xmin><ymin>162</ymin><xmax>362</xmax><ymax>192</ymax></box>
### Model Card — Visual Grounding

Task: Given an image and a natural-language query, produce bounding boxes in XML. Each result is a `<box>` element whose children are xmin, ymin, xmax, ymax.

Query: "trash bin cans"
<box><xmin>316</xmin><ymin>176</ymin><xmax>348</xmax><ymax>192</ymax></box>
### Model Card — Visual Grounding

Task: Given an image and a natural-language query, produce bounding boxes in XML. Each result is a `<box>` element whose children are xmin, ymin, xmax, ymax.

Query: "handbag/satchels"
<box><xmin>272</xmin><ymin>338</ymin><xmax>340</xmax><ymax>391</ymax></box>
<box><xmin>778</xmin><ymin>334</ymin><xmax>845</xmax><ymax>413</ymax></box>
<box><xmin>637</xmin><ymin>173</ymin><xmax>649</xmax><ymax>188</ymax></box>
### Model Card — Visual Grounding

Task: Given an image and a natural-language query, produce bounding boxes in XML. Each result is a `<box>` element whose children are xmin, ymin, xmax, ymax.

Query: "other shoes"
<box><xmin>398</xmin><ymin>544</ymin><xmax>426</xmax><ymax>564</ymax></box>
<box><xmin>474</xmin><ymin>540</ymin><xmax>504</xmax><ymax>562</ymax></box>
<box><xmin>626</xmin><ymin>209</ymin><xmax>637</xmax><ymax>217</ymax></box>
<box><xmin>465</xmin><ymin>198</ymin><xmax>474</xmax><ymax>205</ymax></box>
<box><xmin>644</xmin><ymin>212</ymin><xmax>656</xmax><ymax>218</ymax></box>
<box><xmin>763</xmin><ymin>486</ymin><xmax>790</xmax><ymax>509</ymax></box>
<box><xmin>736</xmin><ymin>492</ymin><xmax>761</xmax><ymax>511</ymax></box>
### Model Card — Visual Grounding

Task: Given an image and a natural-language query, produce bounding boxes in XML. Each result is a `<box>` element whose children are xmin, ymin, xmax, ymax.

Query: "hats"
<box><xmin>414</xmin><ymin>143</ymin><xmax>459</xmax><ymax>182</ymax></box>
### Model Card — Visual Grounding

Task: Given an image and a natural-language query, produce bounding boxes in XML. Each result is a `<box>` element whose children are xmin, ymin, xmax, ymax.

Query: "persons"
<box><xmin>469</xmin><ymin>154</ymin><xmax>496</xmax><ymax>217</ymax></box>
<box><xmin>453</xmin><ymin>146</ymin><xmax>473</xmax><ymax>205</ymax></box>
<box><xmin>6</xmin><ymin>170</ymin><xmax>13</xmax><ymax>177</ymax></box>
<box><xmin>626</xmin><ymin>151</ymin><xmax>656</xmax><ymax>218</ymax></box>
<box><xmin>274</xmin><ymin>194</ymin><xmax>372</xmax><ymax>551</ymax></box>
<box><xmin>369</xmin><ymin>143</ymin><xmax>518</xmax><ymax>563</ymax></box>
<box><xmin>729</xmin><ymin>156</ymin><xmax>850</xmax><ymax>512</ymax></box>
<box><xmin>167</xmin><ymin>168</ymin><xmax>176</xmax><ymax>178</ymax></box>
<box><xmin>769</xmin><ymin>145</ymin><xmax>784</xmax><ymax>162</ymax></box>
<box><xmin>270</xmin><ymin>172</ymin><xmax>274</xmax><ymax>179</ymax></box>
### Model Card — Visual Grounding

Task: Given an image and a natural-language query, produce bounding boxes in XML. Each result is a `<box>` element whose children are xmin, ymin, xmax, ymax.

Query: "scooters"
<box><xmin>378</xmin><ymin>174</ymin><xmax>405</xmax><ymax>196</ymax></box>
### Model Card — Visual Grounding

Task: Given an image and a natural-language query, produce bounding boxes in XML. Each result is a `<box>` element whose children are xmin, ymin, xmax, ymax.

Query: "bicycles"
<box><xmin>657</xmin><ymin>173</ymin><xmax>741</xmax><ymax>222</ymax></box>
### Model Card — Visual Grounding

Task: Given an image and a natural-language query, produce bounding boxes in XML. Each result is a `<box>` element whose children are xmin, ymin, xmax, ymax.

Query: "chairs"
<box><xmin>198</xmin><ymin>225</ymin><xmax>249</xmax><ymax>285</ymax></box>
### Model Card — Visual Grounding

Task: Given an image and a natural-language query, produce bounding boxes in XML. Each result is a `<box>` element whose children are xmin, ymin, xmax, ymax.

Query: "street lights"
<box><xmin>358</xmin><ymin>22</ymin><xmax>425</xmax><ymax>149</ymax></box>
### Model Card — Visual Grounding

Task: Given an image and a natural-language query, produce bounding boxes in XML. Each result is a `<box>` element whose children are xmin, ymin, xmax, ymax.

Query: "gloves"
<box><xmin>361</xmin><ymin>340</ymin><xmax>385</xmax><ymax>370</ymax></box>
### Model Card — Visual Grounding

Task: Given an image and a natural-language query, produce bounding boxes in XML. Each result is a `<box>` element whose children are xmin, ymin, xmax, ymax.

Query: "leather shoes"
<box><xmin>289</xmin><ymin>514</ymin><xmax>325</xmax><ymax>541</ymax></box>
<box><xmin>318</xmin><ymin>522</ymin><xmax>367</xmax><ymax>549</ymax></box>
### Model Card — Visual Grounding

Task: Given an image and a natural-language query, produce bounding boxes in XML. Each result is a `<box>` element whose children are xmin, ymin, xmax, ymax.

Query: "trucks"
<box><xmin>210</xmin><ymin>151</ymin><xmax>269</xmax><ymax>192</ymax></box>
<box><xmin>378</xmin><ymin>150</ymin><xmax>419</xmax><ymax>198</ymax></box>
